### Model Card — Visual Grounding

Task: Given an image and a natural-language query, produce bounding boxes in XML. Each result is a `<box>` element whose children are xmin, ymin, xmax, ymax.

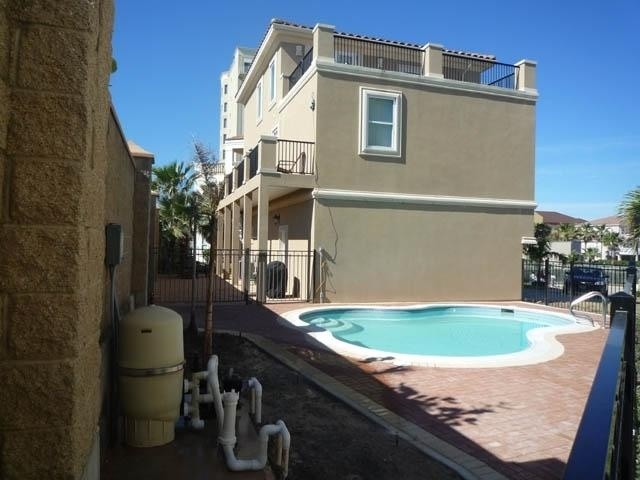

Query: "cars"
<box><xmin>561</xmin><ymin>265</ymin><xmax>608</xmax><ymax>297</ymax></box>
